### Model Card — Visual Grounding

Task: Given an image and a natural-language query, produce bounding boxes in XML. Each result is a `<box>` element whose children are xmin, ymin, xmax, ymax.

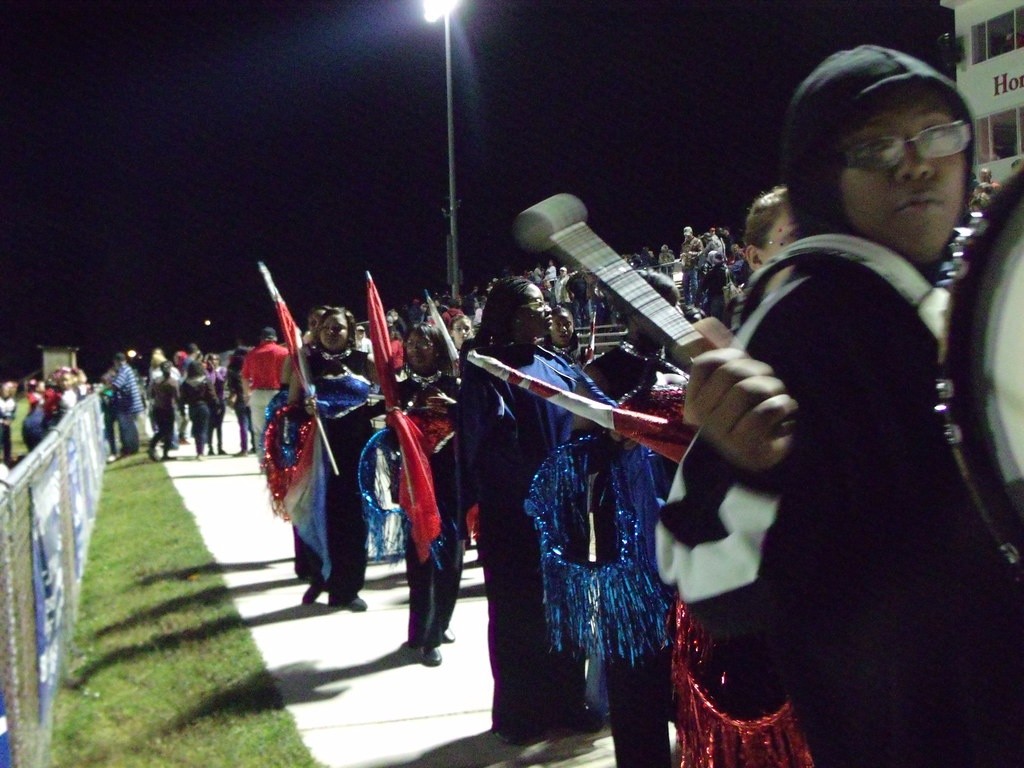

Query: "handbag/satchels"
<box><xmin>722</xmin><ymin>269</ymin><xmax>745</xmax><ymax>309</ymax></box>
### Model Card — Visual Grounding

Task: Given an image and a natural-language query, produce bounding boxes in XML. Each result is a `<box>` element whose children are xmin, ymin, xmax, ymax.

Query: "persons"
<box><xmin>281</xmin><ymin>304</ymin><xmax>377</xmax><ymax>611</ymax></box>
<box><xmin>534</xmin><ymin>300</ymin><xmax>605</xmax><ymax>494</ymax></box>
<box><xmin>0</xmin><ymin>218</ymin><xmax>750</xmax><ymax>462</ymax></box>
<box><xmin>364</xmin><ymin>321</ymin><xmax>466</xmax><ymax>666</ymax></box>
<box><xmin>658</xmin><ymin>186</ymin><xmax>818</xmax><ymax>768</ymax></box>
<box><xmin>970</xmin><ymin>180</ymin><xmax>1000</xmax><ymax>220</ymax></box>
<box><xmin>280</xmin><ymin>307</ymin><xmax>356</xmax><ymax>588</ymax></box>
<box><xmin>975</xmin><ymin>166</ymin><xmax>1003</xmax><ymax>204</ymax></box>
<box><xmin>450</xmin><ymin>269</ymin><xmax>596</xmax><ymax>768</ymax></box>
<box><xmin>641</xmin><ymin>36</ymin><xmax>1023</xmax><ymax>768</ymax></box>
<box><xmin>564</xmin><ymin>268</ymin><xmax>711</xmax><ymax>768</ymax></box>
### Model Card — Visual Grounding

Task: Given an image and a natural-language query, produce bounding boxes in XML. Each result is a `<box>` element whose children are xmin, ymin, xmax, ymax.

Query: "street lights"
<box><xmin>423</xmin><ymin>0</ymin><xmax>462</xmax><ymax>306</ymax></box>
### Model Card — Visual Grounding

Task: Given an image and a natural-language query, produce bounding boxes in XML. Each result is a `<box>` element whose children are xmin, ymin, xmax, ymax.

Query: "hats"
<box><xmin>660</xmin><ymin>244</ymin><xmax>670</xmax><ymax>252</ymax></box>
<box><xmin>559</xmin><ymin>266</ymin><xmax>567</xmax><ymax>272</ymax></box>
<box><xmin>682</xmin><ymin>227</ymin><xmax>694</xmax><ymax>236</ymax></box>
<box><xmin>356</xmin><ymin>325</ymin><xmax>365</xmax><ymax>333</ymax></box>
<box><xmin>261</xmin><ymin>327</ymin><xmax>277</xmax><ymax>342</ymax></box>
<box><xmin>708</xmin><ymin>251</ymin><xmax>727</xmax><ymax>263</ymax></box>
<box><xmin>710</xmin><ymin>227</ymin><xmax>715</xmax><ymax>232</ymax></box>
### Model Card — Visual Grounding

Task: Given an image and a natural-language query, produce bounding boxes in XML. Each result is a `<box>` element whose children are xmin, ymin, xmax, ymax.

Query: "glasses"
<box><xmin>832</xmin><ymin>118</ymin><xmax>977</xmax><ymax>173</ymax></box>
<box><xmin>452</xmin><ymin>327</ymin><xmax>472</xmax><ymax>333</ymax></box>
<box><xmin>318</xmin><ymin>323</ymin><xmax>347</xmax><ymax>334</ymax></box>
<box><xmin>401</xmin><ymin>338</ymin><xmax>435</xmax><ymax>351</ymax></box>
<box><xmin>517</xmin><ymin>299</ymin><xmax>550</xmax><ymax>312</ymax></box>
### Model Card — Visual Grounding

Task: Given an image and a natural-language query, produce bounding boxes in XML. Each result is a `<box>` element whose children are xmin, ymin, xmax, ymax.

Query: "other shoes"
<box><xmin>331</xmin><ymin>595</ymin><xmax>368</xmax><ymax>612</ymax></box>
<box><xmin>248</xmin><ymin>446</ymin><xmax>256</xmax><ymax>454</ymax></box>
<box><xmin>177</xmin><ymin>435</ymin><xmax>190</xmax><ymax>446</ymax></box>
<box><xmin>300</xmin><ymin>584</ymin><xmax>327</xmax><ymax>604</ymax></box>
<box><xmin>206</xmin><ymin>449</ymin><xmax>217</xmax><ymax>457</ymax></box>
<box><xmin>422</xmin><ymin>644</ymin><xmax>445</xmax><ymax>666</ymax></box>
<box><xmin>217</xmin><ymin>447</ymin><xmax>227</xmax><ymax>456</ymax></box>
<box><xmin>233</xmin><ymin>450</ymin><xmax>250</xmax><ymax>457</ymax></box>
<box><xmin>105</xmin><ymin>454</ymin><xmax>119</xmax><ymax>463</ymax></box>
<box><xmin>161</xmin><ymin>454</ymin><xmax>179</xmax><ymax>462</ymax></box>
<box><xmin>439</xmin><ymin>628</ymin><xmax>456</xmax><ymax>642</ymax></box>
<box><xmin>195</xmin><ymin>454</ymin><xmax>205</xmax><ymax>463</ymax></box>
<box><xmin>146</xmin><ymin>448</ymin><xmax>160</xmax><ymax>462</ymax></box>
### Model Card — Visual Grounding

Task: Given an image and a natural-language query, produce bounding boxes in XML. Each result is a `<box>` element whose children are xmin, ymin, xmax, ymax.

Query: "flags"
<box><xmin>366</xmin><ymin>274</ymin><xmax>442</xmax><ymax>561</ymax></box>
